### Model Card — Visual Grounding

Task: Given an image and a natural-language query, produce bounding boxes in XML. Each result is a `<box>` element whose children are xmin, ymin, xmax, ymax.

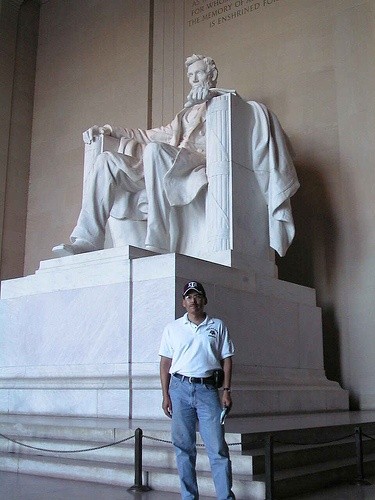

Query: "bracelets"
<box><xmin>223</xmin><ymin>387</ymin><xmax>232</xmax><ymax>392</ymax></box>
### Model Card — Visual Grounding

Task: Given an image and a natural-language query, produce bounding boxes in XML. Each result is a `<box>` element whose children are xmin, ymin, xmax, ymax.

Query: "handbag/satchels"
<box><xmin>212</xmin><ymin>369</ymin><xmax>224</xmax><ymax>388</ymax></box>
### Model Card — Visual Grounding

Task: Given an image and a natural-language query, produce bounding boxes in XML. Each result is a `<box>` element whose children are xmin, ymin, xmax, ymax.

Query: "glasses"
<box><xmin>185</xmin><ymin>295</ymin><xmax>203</xmax><ymax>301</ymax></box>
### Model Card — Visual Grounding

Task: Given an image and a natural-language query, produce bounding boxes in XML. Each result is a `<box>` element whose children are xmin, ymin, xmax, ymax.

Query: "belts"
<box><xmin>173</xmin><ymin>373</ymin><xmax>212</xmax><ymax>384</ymax></box>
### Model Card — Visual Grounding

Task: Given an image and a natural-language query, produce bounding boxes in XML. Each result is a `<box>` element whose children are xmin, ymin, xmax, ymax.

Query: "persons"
<box><xmin>157</xmin><ymin>281</ymin><xmax>235</xmax><ymax>500</ymax></box>
<box><xmin>52</xmin><ymin>53</ymin><xmax>237</xmax><ymax>257</ymax></box>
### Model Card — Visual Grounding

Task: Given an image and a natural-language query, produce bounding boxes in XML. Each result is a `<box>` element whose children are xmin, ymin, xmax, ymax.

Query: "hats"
<box><xmin>182</xmin><ymin>280</ymin><xmax>205</xmax><ymax>295</ymax></box>
